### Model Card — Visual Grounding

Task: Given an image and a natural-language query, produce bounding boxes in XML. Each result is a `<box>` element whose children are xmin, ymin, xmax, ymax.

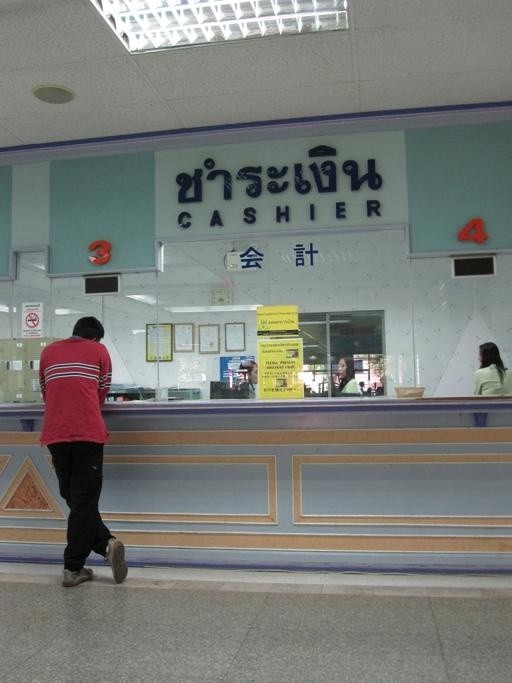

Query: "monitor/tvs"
<box><xmin>210</xmin><ymin>381</ymin><xmax>249</xmax><ymax>399</ymax></box>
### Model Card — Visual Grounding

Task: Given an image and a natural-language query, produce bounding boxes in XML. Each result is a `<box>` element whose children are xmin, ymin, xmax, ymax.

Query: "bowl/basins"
<box><xmin>393</xmin><ymin>386</ymin><xmax>425</xmax><ymax>397</ymax></box>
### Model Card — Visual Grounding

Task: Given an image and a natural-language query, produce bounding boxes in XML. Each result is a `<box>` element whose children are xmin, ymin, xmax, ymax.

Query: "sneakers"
<box><xmin>105</xmin><ymin>537</ymin><xmax>129</xmax><ymax>585</ymax></box>
<box><xmin>61</xmin><ymin>566</ymin><xmax>94</xmax><ymax>589</ymax></box>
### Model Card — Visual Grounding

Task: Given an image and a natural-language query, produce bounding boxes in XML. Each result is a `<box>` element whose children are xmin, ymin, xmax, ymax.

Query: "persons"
<box><xmin>238</xmin><ymin>361</ymin><xmax>258</xmax><ymax>398</ymax></box>
<box><xmin>38</xmin><ymin>317</ymin><xmax>126</xmax><ymax>588</ymax></box>
<box><xmin>338</xmin><ymin>357</ymin><xmax>359</xmax><ymax>393</ymax></box>
<box><xmin>473</xmin><ymin>342</ymin><xmax>512</xmax><ymax>395</ymax></box>
